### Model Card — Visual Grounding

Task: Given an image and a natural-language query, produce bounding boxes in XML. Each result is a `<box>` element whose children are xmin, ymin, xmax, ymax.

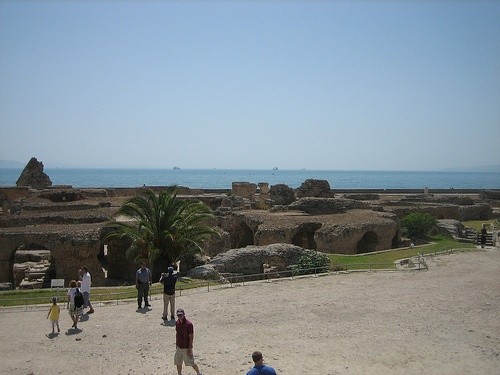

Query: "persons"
<box><xmin>173</xmin><ymin>308</ymin><xmax>202</xmax><ymax>375</ymax></box>
<box><xmin>135</xmin><ymin>260</ymin><xmax>153</xmax><ymax>311</ymax></box>
<box><xmin>159</xmin><ymin>261</ymin><xmax>180</xmax><ymax>326</ymax></box>
<box><xmin>66</xmin><ymin>280</ymin><xmax>84</xmax><ymax>329</ymax></box>
<box><xmin>246</xmin><ymin>351</ymin><xmax>276</xmax><ymax>375</ymax></box>
<box><xmin>76</xmin><ymin>265</ymin><xmax>94</xmax><ymax>316</ymax></box>
<box><xmin>47</xmin><ymin>296</ymin><xmax>60</xmax><ymax>334</ymax></box>
<box><xmin>480</xmin><ymin>223</ymin><xmax>488</xmax><ymax>249</ymax></box>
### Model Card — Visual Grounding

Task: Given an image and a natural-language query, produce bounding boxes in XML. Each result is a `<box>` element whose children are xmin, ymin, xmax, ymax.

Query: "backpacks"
<box><xmin>74</xmin><ymin>288</ymin><xmax>84</xmax><ymax>308</ymax></box>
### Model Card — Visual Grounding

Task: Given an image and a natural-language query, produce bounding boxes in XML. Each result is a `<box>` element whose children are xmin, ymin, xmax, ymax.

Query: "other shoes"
<box><xmin>72</xmin><ymin>323</ymin><xmax>77</xmax><ymax>329</ymax></box>
<box><xmin>139</xmin><ymin>305</ymin><xmax>142</xmax><ymax>308</ymax></box>
<box><xmin>87</xmin><ymin>309</ymin><xmax>94</xmax><ymax>313</ymax></box>
<box><xmin>171</xmin><ymin>316</ymin><xmax>175</xmax><ymax>319</ymax></box>
<box><xmin>162</xmin><ymin>316</ymin><xmax>167</xmax><ymax>320</ymax></box>
<box><xmin>145</xmin><ymin>304</ymin><xmax>151</xmax><ymax>306</ymax></box>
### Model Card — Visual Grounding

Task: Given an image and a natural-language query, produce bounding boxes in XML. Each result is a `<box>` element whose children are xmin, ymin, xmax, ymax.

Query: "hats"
<box><xmin>177</xmin><ymin>308</ymin><xmax>184</xmax><ymax>315</ymax></box>
